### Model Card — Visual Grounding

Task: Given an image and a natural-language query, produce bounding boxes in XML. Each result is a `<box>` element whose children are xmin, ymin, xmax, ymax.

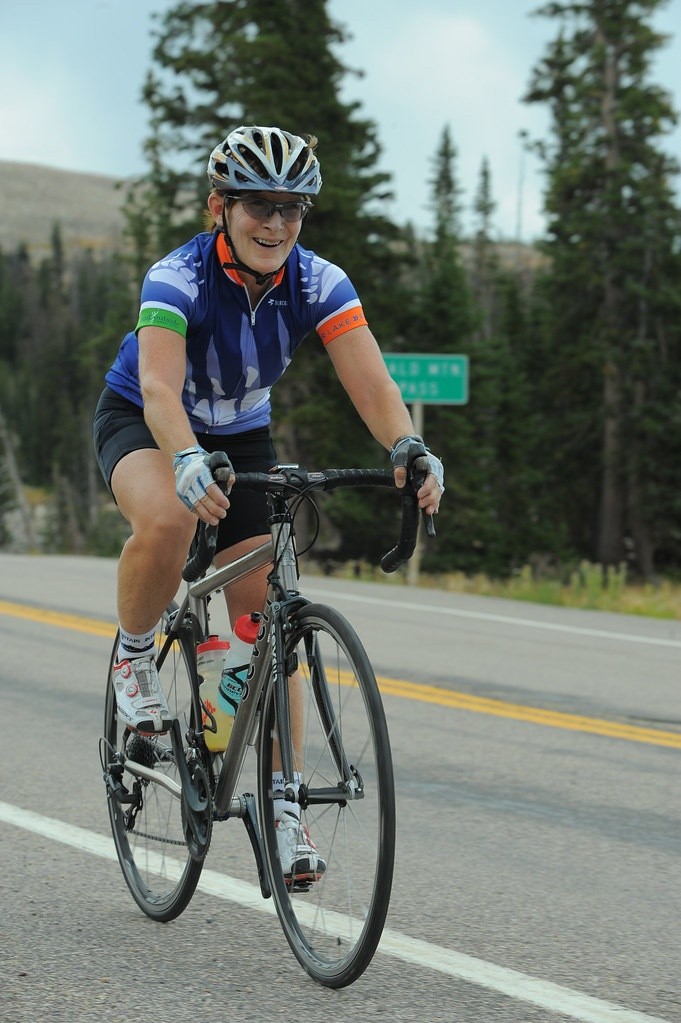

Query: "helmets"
<box><xmin>207</xmin><ymin>127</ymin><xmax>323</xmax><ymax>195</ymax></box>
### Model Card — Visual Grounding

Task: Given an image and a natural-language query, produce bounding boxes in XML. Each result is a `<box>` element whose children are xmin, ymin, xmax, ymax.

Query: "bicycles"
<box><xmin>100</xmin><ymin>443</ymin><xmax>436</xmax><ymax>990</ymax></box>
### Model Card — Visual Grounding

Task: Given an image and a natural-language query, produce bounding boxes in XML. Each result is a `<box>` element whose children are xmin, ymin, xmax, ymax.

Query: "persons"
<box><xmin>84</xmin><ymin>121</ymin><xmax>447</xmax><ymax>893</ymax></box>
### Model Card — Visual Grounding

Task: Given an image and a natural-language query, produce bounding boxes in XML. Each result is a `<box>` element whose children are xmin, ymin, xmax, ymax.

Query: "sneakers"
<box><xmin>110</xmin><ymin>654</ymin><xmax>173</xmax><ymax>736</ymax></box>
<box><xmin>273</xmin><ymin>810</ymin><xmax>326</xmax><ymax>883</ymax></box>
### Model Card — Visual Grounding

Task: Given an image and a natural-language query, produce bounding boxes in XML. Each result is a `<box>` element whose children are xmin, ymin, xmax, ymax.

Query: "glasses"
<box><xmin>220</xmin><ymin>190</ymin><xmax>313</xmax><ymax>222</ymax></box>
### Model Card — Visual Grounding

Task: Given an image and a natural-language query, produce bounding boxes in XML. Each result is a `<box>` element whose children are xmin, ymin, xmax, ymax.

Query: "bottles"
<box><xmin>215</xmin><ymin>611</ymin><xmax>261</xmax><ymax>716</ymax></box>
<box><xmin>196</xmin><ymin>633</ymin><xmax>236</xmax><ymax>752</ymax></box>
